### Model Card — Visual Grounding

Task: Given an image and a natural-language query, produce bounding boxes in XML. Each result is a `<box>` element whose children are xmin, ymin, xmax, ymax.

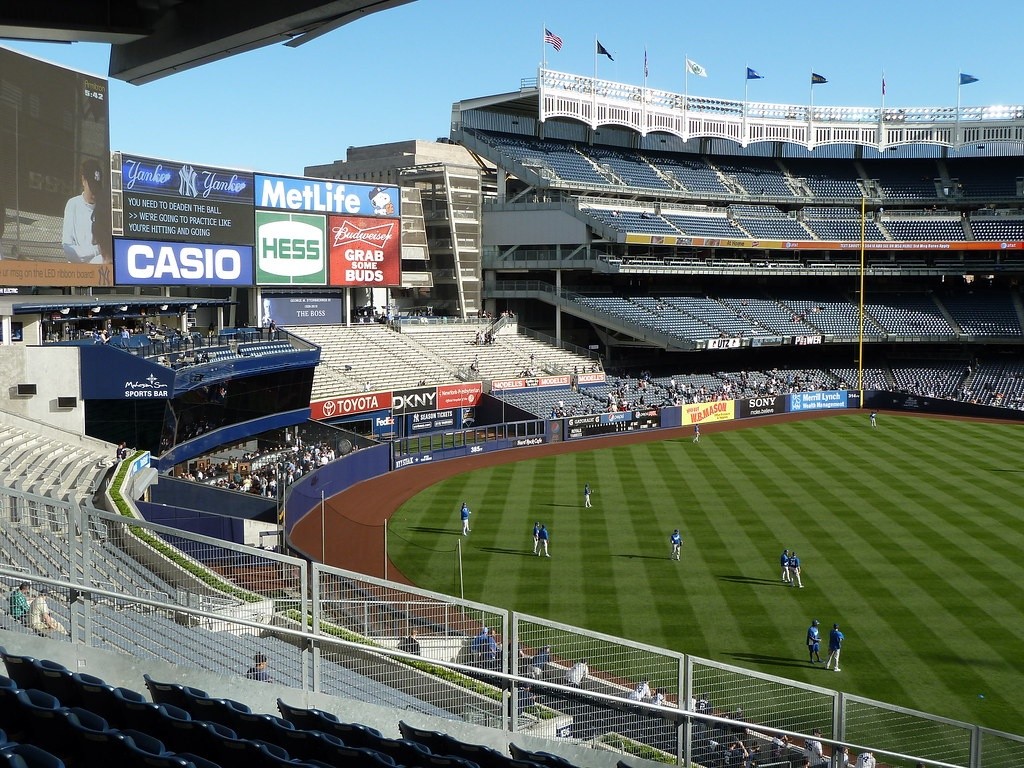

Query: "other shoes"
<box><xmin>825</xmin><ymin>666</ymin><xmax>829</xmax><ymax>669</ymax></box>
<box><xmin>468</xmin><ymin>530</ymin><xmax>471</xmax><ymax>531</ymax></box>
<box><xmin>533</xmin><ymin>549</ymin><xmax>536</xmax><ymax>554</ymax></box>
<box><xmin>586</xmin><ymin>506</ymin><xmax>593</xmax><ymax>508</ymax></box>
<box><xmin>545</xmin><ymin>553</ymin><xmax>551</xmax><ymax>557</ymax></box>
<box><xmin>817</xmin><ymin>659</ymin><xmax>823</xmax><ymax>663</ymax></box>
<box><xmin>799</xmin><ymin>586</ymin><xmax>804</xmax><ymax>588</ymax></box>
<box><xmin>810</xmin><ymin>659</ymin><xmax>814</xmax><ymax>664</ymax></box>
<box><xmin>538</xmin><ymin>552</ymin><xmax>540</xmax><ymax>556</ymax></box>
<box><xmin>461</xmin><ymin>532</ymin><xmax>466</xmax><ymax>535</ymax></box>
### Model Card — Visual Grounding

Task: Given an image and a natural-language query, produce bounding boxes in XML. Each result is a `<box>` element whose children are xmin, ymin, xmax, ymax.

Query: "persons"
<box><xmin>806</xmin><ymin>618</ymin><xmax>844</xmax><ymax>672</ymax></box>
<box><xmin>61</xmin><ymin>159</ymin><xmax>112</xmax><ymax>265</ymax></box>
<box><xmin>916</xmin><ymin>763</ymin><xmax>926</xmax><ymax>768</ymax></box>
<box><xmin>116</xmin><ymin>388</ymin><xmax>361</xmax><ymax>499</ymax></box>
<box><xmin>623</xmin><ymin>675</ymin><xmax>876</xmax><ymax>768</ymax></box>
<box><xmin>37</xmin><ymin>318</ymin><xmax>279</xmax><ymax>369</ymax></box>
<box><xmin>246</xmin><ymin>652</ymin><xmax>273</xmax><ymax>683</ymax></box>
<box><xmin>868</xmin><ymin>411</ymin><xmax>876</xmax><ymax>428</ymax></box>
<box><xmin>404</xmin><ymin>626</ymin><xmax>590</xmax><ymax>716</ymax></box>
<box><xmin>9</xmin><ymin>582</ymin><xmax>71</xmax><ymax>636</ymax></box>
<box><xmin>532</xmin><ymin>522</ymin><xmax>550</xmax><ymax>557</ymax></box>
<box><xmin>584</xmin><ymin>483</ymin><xmax>594</xmax><ymax>508</ymax></box>
<box><xmin>692</xmin><ymin>423</ymin><xmax>700</xmax><ymax>443</ymax></box>
<box><xmin>780</xmin><ymin>549</ymin><xmax>805</xmax><ymax>587</ymax></box>
<box><xmin>670</xmin><ymin>529</ymin><xmax>684</xmax><ymax>561</ymax></box>
<box><xmin>350</xmin><ymin>294</ymin><xmax>1024</xmax><ymax>420</ymax></box>
<box><xmin>461</xmin><ymin>503</ymin><xmax>472</xmax><ymax>537</ymax></box>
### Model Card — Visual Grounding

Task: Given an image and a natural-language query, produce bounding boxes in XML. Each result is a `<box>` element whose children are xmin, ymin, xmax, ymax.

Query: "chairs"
<box><xmin>0</xmin><ymin>131</ymin><xmax>1024</xmax><ymax>768</ymax></box>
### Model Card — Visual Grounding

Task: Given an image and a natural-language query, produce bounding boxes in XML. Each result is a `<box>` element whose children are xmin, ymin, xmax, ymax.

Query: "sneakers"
<box><xmin>834</xmin><ymin>667</ymin><xmax>841</xmax><ymax>671</ymax></box>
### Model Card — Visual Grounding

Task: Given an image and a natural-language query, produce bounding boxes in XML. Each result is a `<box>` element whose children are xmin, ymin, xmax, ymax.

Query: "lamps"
<box><xmin>189</xmin><ymin>304</ymin><xmax>198</xmax><ymax>310</ymax></box>
<box><xmin>60</xmin><ymin>307</ymin><xmax>70</xmax><ymax>314</ymax></box>
<box><xmin>117</xmin><ymin>306</ymin><xmax>128</xmax><ymax>311</ymax></box>
<box><xmin>91</xmin><ymin>306</ymin><xmax>101</xmax><ymax>313</ymax></box>
<box><xmin>159</xmin><ymin>304</ymin><xmax>168</xmax><ymax>311</ymax></box>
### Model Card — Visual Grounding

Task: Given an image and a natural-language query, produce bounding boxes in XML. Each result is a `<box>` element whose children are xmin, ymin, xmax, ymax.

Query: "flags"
<box><xmin>542</xmin><ymin>29</ymin><xmax>980</xmax><ymax>96</ymax></box>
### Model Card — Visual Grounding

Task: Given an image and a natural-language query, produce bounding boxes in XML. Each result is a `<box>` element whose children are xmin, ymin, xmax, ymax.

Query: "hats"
<box><xmin>535</xmin><ymin>522</ymin><xmax>540</xmax><ymax>525</ymax></box>
<box><xmin>784</xmin><ymin>550</ymin><xmax>789</xmax><ymax>552</ymax></box>
<box><xmin>542</xmin><ymin>524</ymin><xmax>545</xmax><ymax>528</ymax></box>
<box><xmin>792</xmin><ymin>552</ymin><xmax>796</xmax><ymax>556</ymax></box>
<box><xmin>81</xmin><ymin>159</ymin><xmax>103</xmax><ymax>193</ymax></box>
<box><xmin>585</xmin><ymin>484</ymin><xmax>588</xmax><ymax>487</ymax></box>
<box><xmin>463</xmin><ymin>502</ymin><xmax>466</xmax><ymax>505</ymax></box>
<box><xmin>834</xmin><ymin>623</ymin><xmax>839</xmax><ymax>626</ymax></box>
<box><xmin>812</xmin><ymin>619</ymin><xmax>820</xmax><ymax>625</ymax></box>
<box><xmin>674</xmin><ymin>529</ymin><xmax>679</xmax><ymax>532</ymax></box>
